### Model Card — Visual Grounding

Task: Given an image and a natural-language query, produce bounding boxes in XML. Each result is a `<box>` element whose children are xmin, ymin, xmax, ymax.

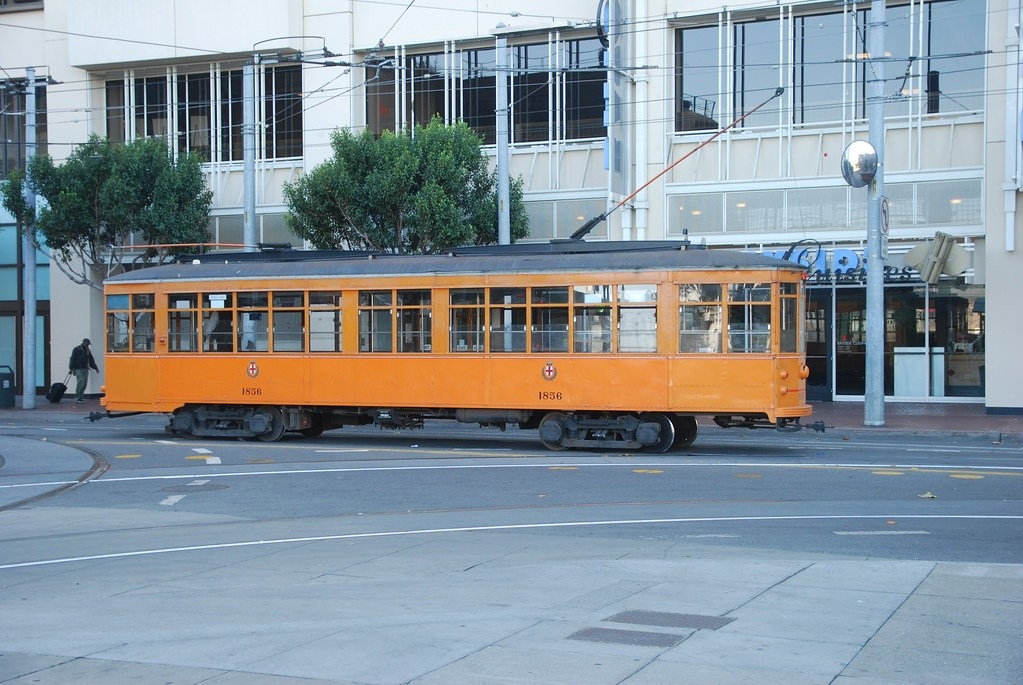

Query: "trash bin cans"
<box><xmin>0</xmin><ymin>365</ymin><xmax>15</xmax><ymax>408</ymax></box>
<box><xmin>979</xmin><ymin>365</ymin><xmax>985</xmax><ymax>387</ymax></box>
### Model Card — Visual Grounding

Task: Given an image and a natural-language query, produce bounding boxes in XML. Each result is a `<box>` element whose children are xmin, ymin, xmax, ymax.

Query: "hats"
<box><xmin>83</xmin><ymin>338</ymin><xmax>91</xmax><ymax>345</ymax></box>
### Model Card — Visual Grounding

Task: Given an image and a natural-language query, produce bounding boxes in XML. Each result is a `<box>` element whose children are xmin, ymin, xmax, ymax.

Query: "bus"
<box><xmin>80</xmin><ymin>89</ymin><xmax>834</xmax><ymax>452</ymax></box>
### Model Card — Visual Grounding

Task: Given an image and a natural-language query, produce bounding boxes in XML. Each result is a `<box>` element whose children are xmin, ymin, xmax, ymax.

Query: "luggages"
<box><xmin>46</xmin><ymin>371</ymin><xmax>73</xmax><ymax>404</ymax></box>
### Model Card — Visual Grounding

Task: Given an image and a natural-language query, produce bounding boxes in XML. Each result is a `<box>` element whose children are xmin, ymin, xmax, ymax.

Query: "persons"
<box><xmin>203</xmin><ymin>311</ymin><xmax>233</xmax><ymax>352</ymax></box>
<box><xmin>69</xmin><ymin>338</ymin><xmax>99</xmax><ymax>403</ymax></box>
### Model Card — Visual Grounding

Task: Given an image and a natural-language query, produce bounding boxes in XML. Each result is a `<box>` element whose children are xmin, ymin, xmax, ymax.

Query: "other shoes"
<box><xmin>75</xmin><ymin>398</ymin><xmax>87</xmax><ymax>404</ymax></box>
